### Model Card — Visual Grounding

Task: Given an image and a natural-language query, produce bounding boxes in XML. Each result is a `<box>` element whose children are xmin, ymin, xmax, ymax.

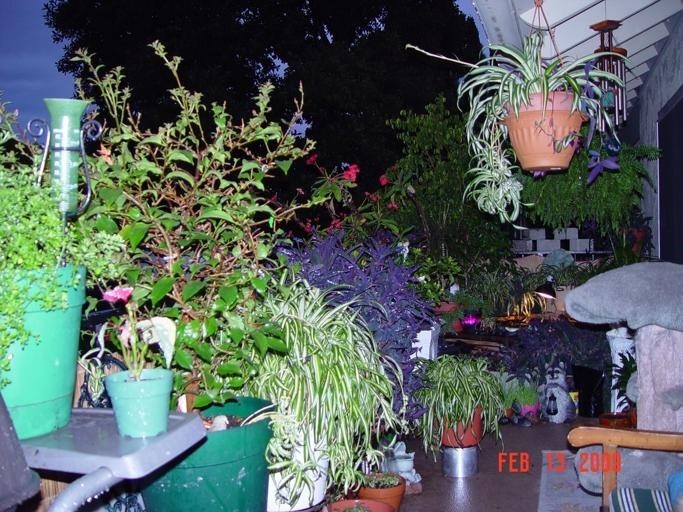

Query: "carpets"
<box><xmin>536</xmin><ymin>447</ymin><xmax>608</xmax><ymax>511</ymax></box>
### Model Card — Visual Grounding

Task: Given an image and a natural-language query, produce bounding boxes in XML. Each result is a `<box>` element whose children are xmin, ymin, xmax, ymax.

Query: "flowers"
<box><xmin>90</xmin><ymin>279</ymin><xmax>180</xmax><ymax>379</ymax></box>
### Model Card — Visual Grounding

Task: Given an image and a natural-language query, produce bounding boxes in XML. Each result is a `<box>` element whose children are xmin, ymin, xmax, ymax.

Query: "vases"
<box><xmin>597</xmin><ymin>402</ymin><xmax>637</xmax><ymax>427</ymax></box>
<box><xmin>102</xmin><ymin>367</ymin><xmax>173</xmax><ymax>438</ymax></box>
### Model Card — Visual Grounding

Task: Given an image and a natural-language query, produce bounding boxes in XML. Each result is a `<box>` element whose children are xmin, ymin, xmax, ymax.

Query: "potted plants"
<box><xmin>388</xmin><ymin>100</ymin><xmax>493</xmax><ymax>333</ymax></box>
<box><xmin>252</xmin><ymin>280</ymin><xmax>407</xmax><ymax>512</ymax></box>
<box><xmin>327</xmin><ymin>498</ymin><xmax>394</xmax><ymax>512</ymax></box>
<box><xmin>455</xmin><ymin>38</ymin><xmax>634</xmax><ymax>232</ymax></box>
<box><xmin>413</xmin><ymin>353</ymin><xmax>503</xmax><ymax>461</ymax></box>
<box><xmin>538</xmin><ymin>259</ymin><xmax>582</xmax><ymax>313</ymax></box>
<box><xmin>354</xmin><ymin>468</ymin><xmax>412</xmax><ymax>505</ymax></box>
<box><xmin>0</xmin><ymin>98</ymin><xmax>116</xmax><ymax>443</ymax></box>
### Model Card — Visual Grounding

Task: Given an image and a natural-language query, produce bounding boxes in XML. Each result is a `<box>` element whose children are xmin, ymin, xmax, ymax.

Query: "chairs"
<box><xmin>566</xmin><ymin>425</ymin><xmax>683</xmax><ymax>511</ymax></box>
